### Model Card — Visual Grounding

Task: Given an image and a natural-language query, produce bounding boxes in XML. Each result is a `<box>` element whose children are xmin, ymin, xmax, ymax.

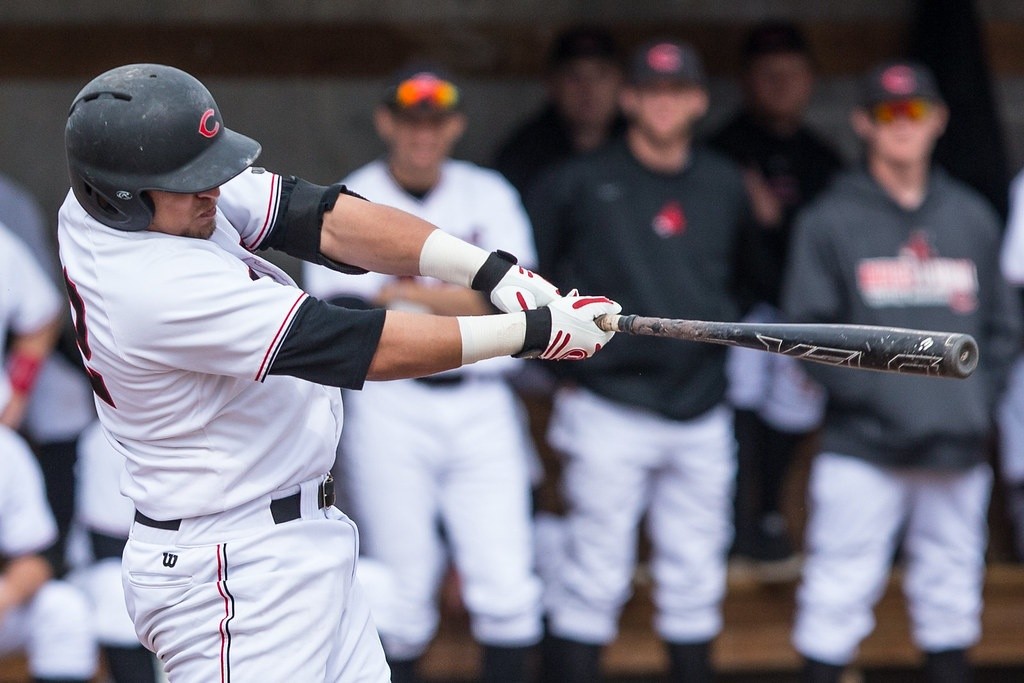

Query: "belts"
<box><xmin>416</xmin><ymin>373</ymin><xmax>462</xmax><ymax>387</ymax></box>
<box><xmin>133</xmin><ymin>469</ymin><xmax>334</xmax><ymax>530</ymax></box>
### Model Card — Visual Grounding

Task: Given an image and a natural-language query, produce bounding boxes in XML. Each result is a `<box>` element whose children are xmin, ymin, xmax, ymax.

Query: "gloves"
<box><xmin>472</xmin><ymin>248</ymin><xmax>564</xmax><ymax>313</ymax></box>
<box><xmin>510</xmin><ymin>295</ymin><xmax>622</xmax><ymax>359</ymax></box>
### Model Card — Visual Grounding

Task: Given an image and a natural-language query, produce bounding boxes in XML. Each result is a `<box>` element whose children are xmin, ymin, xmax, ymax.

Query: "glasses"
<box><xmin>868</xmin><ymin>96</ymin><xmax>933</xmax><ymax>126</ymax></box>
<box><xmin>391</xmin><ymin>79</ymin><xmax>460</xmax><ymax>114</ymax></box>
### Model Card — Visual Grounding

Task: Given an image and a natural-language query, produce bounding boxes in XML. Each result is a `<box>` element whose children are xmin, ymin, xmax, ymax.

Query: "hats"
<box><xmin>544</xmin><ymin>25</ymin><xmax>627</xmax><ymax>72</ymax></box>
<box><xmin>382</xmin><ymin>54</ymin><xmax>468</xmax><ymax>125</ymax></box>
<box><xmin>624</xmin><ymin>37</ymin><xmax>708</xmax><ymax>94</ymax></box>
<box><xmin>738</xmin><ymin>21</ymin><xmax>825</xmax><ymax>73</ymax></box>
<box><xmin>852</xmin><ymin>54</ymin><xmax>942</xmax><ymax>107</ymax></box>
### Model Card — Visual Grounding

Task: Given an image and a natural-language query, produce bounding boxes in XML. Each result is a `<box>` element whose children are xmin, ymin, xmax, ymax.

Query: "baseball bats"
<box><xmin>595</xmin><ymin>314</ymin><xmax>979</xmax><ymax>379</ymax></box>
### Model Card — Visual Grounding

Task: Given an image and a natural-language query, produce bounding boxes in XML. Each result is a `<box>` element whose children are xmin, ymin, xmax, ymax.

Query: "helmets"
<box><xmin>63</xmin><ymin>62</ymin><xmax>260</xmax><ymax>232</ymax></box>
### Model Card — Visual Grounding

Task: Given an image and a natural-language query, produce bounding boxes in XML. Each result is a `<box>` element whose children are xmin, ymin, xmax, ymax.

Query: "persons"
<box><xmin>491</xmin><ymin>18</ymin><xmax>635</xmax><ymax>271</ymax></box>
<box><xmin>688</xmin><ymin>22</ymin><xmax>853</xmax><ymax>565</ymax></box>
<box><xmin>300</xmin><ymin>61</ymin><xmax>555</xmax><ymax>683</ymax></box>
<box><xmin>0</xmin><ymin>170</ymin><xmax>161</xmax><ymax>683</ymax></box>
<box><xmin>785</xmin><ymin>48</ymin><xmax>1023</xmax><ymax>683</ymax></box>
<box><xmin>526</xmin><ymin>39</ymin><xmax>792</xmax><ymax>683</ymax></box>
<box><xmin>50</xmin><ymin>59</ymin><xmax>624</xmax><ymax>683</ymax></box>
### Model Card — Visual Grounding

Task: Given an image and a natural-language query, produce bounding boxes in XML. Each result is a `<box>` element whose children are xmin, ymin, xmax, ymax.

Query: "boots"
<box><xmin>543</xmin><ymin>634</ymin><xmax>601</xmax><ymax>683</ymax></box>
<box><xmin>923</xmin><ymin>649</ymin><xmax>966</xmax><ymax>683</ymax></box>
<box><xmin>665</xmin><ymin>643</ymin><xmax>711</xmax><ymax>683</ymax></box>
<box><xmin>387</xmin><ymin>657</ymin><xmax>415</xmax><ymax>683</ymax></box>
<box><xmin>801</xmin><ymin>660</ymin><xmax>842</xmax><ymax>683</ymax></box>
<box><xmin>749</xmin><ymin>419</ymin><xmax>809</xmax><ymax>599</ymax></box>
<box><xmin>479</xmin><ymin>644</ymin><xmax>543</xmax><ymax>683</ymax></box>
<box><xmin>105</xmin><ymin>643</ymin><xmax>155</xmax><ymax>683</ymax></box>
<box><xmin>727</xmin><ymin>402</ymin><xmax>763</xmax><ymax>596</ymax></box>
